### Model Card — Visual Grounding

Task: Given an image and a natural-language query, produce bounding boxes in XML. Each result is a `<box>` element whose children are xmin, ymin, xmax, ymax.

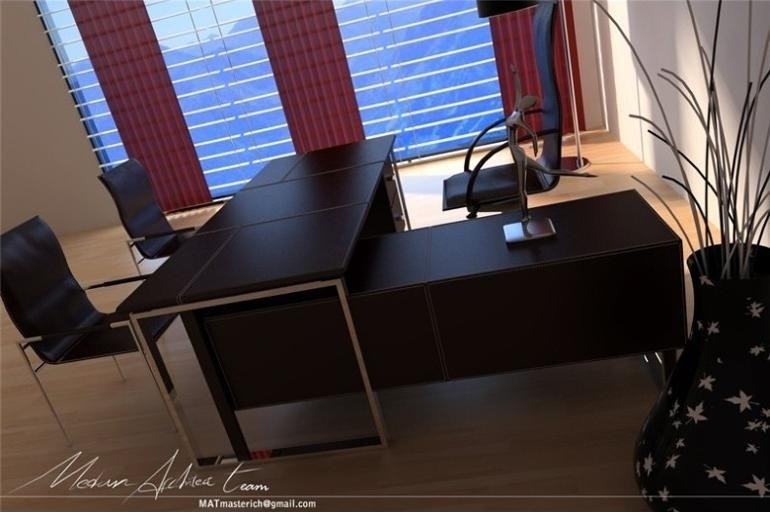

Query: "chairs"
<box><xmin>442</xmin><ymin>1</ymin><xmax>564</xmax><ymax>220</ymax></box>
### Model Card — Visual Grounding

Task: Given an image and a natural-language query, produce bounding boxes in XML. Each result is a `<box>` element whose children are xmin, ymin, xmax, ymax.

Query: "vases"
<box><xmin>635</xmin><ymin>243</ymin><xmax>770</xmax><ymax>512</ymax></box>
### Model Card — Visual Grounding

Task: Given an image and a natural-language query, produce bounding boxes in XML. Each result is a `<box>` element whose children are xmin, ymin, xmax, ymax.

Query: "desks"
<box><xmin>102</xmin><ymin>133</ymin><xmax>688</xmax><ymax>469</ymax></box>
